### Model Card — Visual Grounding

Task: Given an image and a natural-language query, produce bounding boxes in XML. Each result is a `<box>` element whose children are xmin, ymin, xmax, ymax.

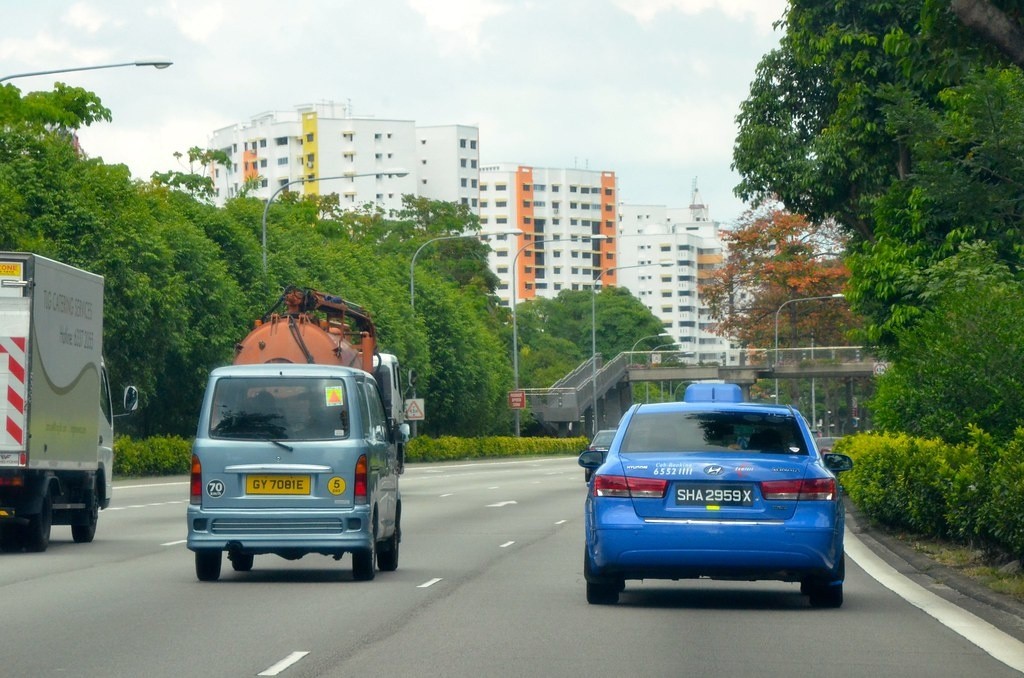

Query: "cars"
<box><xmin>584</xmin><ymin>427</ymin><xmax>620</xmax><ymax>483</ymax></box>
<box><xmin>576</xmin><ymin>382</ymin><xmax>853</xmax><ymax>607</ymax></box>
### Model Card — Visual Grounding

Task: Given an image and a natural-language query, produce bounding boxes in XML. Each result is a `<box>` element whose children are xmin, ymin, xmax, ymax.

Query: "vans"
<box><xmin>186</xmin><ymin>363</ymin><xmax>411</xmax><ymax>581</ymax></box>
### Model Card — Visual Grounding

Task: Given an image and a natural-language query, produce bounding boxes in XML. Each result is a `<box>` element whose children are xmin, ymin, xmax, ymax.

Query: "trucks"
<box><xmin>1</xmin><ymin>250</ymin><xmax>139</xmax><ymax>554</ymax></box>
<box><xmin>230</xmin><ymin>283</ymin><xmax>417</xmax><ymax>476</ymax></box>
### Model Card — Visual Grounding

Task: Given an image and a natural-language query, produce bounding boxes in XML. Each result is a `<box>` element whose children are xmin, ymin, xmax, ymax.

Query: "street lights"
<box><xmin>409</xmin><ymin>227</ymin><xmax>525</xmax><ymax>440</ymax></box>
<box><xmin>511</xmin><ymin>233</ymin><xmax>609</xmax><ymax>439</ymax></box>
<box><xmin>591</xmin><ymin>261</ymin><xmax>675</xmax><ymax>441</ymax></box>
<box><xmin>262</xmin><ymin>171</ymin><xmax>411</xmax><ymax>271</ymax></box>
<box><xmin>630</xmin><ymin>332</ymin><xmax>697</xmax><ymax>406</ymax></box>
<box><xmin>774</xmin><ymin>294</ymin><xmax>847</xmax><ymax>407</ymax></box>
